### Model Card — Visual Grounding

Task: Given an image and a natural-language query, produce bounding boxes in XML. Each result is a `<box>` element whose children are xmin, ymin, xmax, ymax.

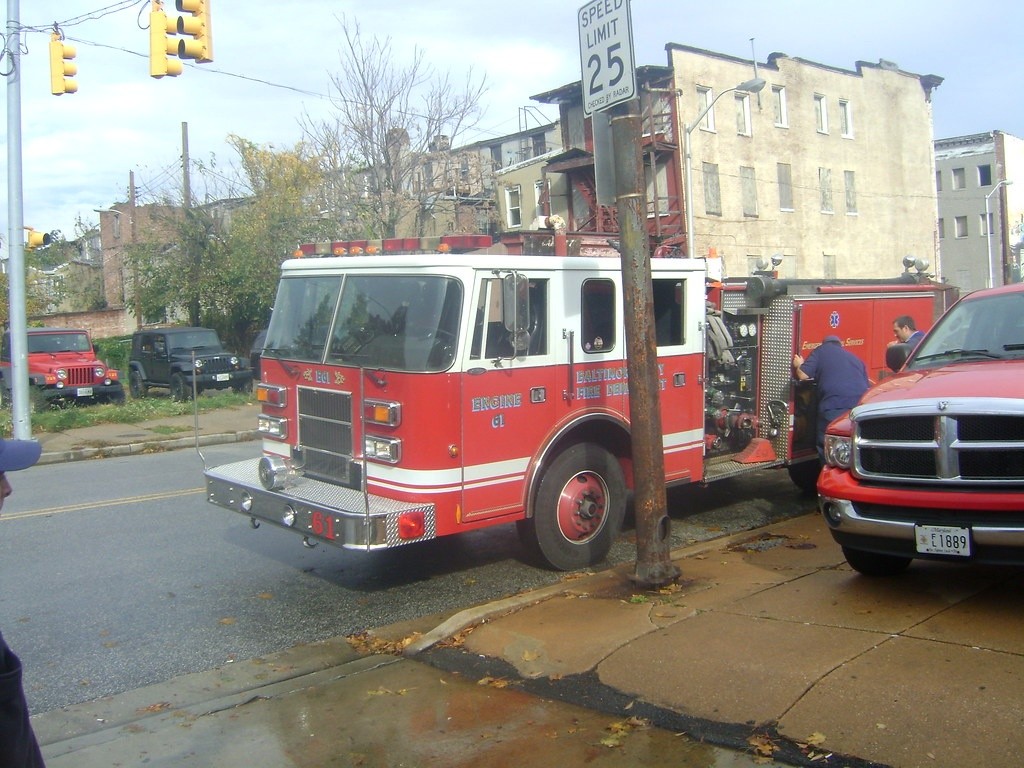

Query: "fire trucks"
<box><xmin>191</xmin><ymin>214</ymin><xmax>942</xmax><ymax>573</ymax></box>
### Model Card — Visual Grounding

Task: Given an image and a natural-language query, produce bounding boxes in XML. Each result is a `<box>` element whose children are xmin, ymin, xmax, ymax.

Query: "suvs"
<box><xmin>814</xmin><ymin>281</ymin><xmax>1024</xmax><ymax>598</ymax></box>
<box><xmin>126</xmin><ymin>326</ymin><xmax>255</xmax><ymax>403</ymax></box>
<box><xmin>0</xmin><ymin>326</ymin><xmax>126</xmax><ymax>412</ymax></box>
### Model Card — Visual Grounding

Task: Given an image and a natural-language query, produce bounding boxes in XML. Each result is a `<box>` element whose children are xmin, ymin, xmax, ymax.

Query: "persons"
<box><xmin>0</xmin><ymin>439</ymin><xmax>45</xmax><ymax>768</ymax></box>
<box><xmin>887</xmin><ymin>316</ymin><xmax>926</xmax><ymax>350</ymax></box>
<box><xmin>793</xmin><ymin>335</ymin><xmax>869</xmax><ymax>468</ymax></box>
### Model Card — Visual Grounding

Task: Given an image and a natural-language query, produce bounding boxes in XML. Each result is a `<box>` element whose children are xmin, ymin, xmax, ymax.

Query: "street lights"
<box><xmin>91</xmin><ymin>207</ymin><xmax>142</xmax><ymax>330</ymax></box>
<box><xmin>984</xmin><ymin>179</ymin><xmax>1016</xmax><ymax>288</ymax></box>
<box><xmin>683</xmin><ymin>75</ymin><xmax>773</xmax><ymax>261</ymax></box>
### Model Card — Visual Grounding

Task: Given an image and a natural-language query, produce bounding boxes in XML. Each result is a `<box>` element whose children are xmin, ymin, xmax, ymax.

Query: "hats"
<box><xmin>0</xmin><ymin>435</ymin><xmax>42</xmax><ymax>472</ymax></box>
<box><xmin>823</xmin><ymin>335</ymin><xmax>839</xmax><ymax>342</ymax></box>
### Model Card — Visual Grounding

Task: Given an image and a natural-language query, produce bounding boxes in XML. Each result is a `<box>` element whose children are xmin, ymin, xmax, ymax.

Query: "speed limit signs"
<box><xmin>576</xmin><ymin>0</ymin><xmax>639</xmax><ymax>119</ymax></box>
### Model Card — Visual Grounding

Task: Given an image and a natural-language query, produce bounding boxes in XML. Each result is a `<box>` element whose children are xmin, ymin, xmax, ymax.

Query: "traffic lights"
<box><xmin>28</xmin><ymin>230</ymin><xmax>51</xmax><ymax>249</ymax></box>
<box><xmin>148</xmin><ymin>11</ymin><xmax>183</xmax><ymax>80</ymax></box>
<box><xmin>48</xmin><ymin>34</ymin><xmax>79</xmax><ymax>96</ymax></box>
<box><xmin>174</xmin><ymin>0</ymin><xmax>214</xmax><ymax>64</ymax></box>
